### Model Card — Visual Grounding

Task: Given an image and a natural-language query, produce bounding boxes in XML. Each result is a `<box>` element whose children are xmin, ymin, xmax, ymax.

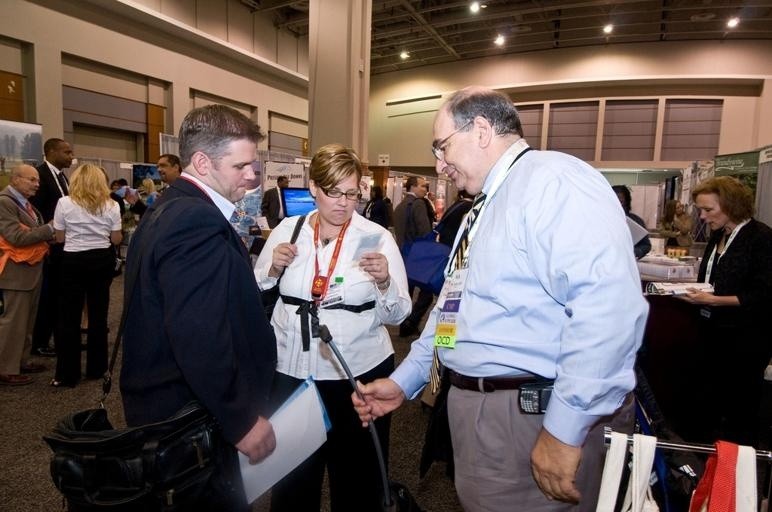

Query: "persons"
<box><xmin>351</xmin><ymin>85</ymin><xmax>651</xmax><ymax>512</ymax></box>
<box><xmin>118</xmin><ymin>104</ymin><xmax>278</xmax><ymax>512</ymax></box>
<box><xmin>253</xmin><ymin>143</ymin><xmax>413</xmax><ymax>511</ymax></box>
<box><xmin>611</xmin><ymin>185</ymin><xmax>651</xmax><ymax>292</ymax></box>
<box><xmin>671</xmin><ymin>175</ymin><xmax>770</xmax><ymax>444</ymax></box>
<box><xmin>362</xmin><ymin>176</ymin><xmax>475</xmax><ymax>337</ymax></box>
<box><xmin>656</xmin><ymin>199</ymin><xmax>693</xmax><ymax>255</ymax></box>
<box><xmin>261</xmin><ymin>176</ymin><xmax>289</xmax><ymax>228</ymax></box>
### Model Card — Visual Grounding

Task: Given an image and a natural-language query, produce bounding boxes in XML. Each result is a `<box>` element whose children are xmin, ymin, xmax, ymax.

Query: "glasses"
<box><xmin>432</xmin><ymin>120</ymin><xmax>472</xmax><ymax>161</ymax></box>
<box><xmin>320</xmin><ymin>186</ymin><xmax>360</xmax><ymax>200</ymax></box>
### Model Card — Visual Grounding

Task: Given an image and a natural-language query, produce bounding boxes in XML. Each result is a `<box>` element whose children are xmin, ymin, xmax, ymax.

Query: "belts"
<box><xmin>445</xmin><ymin>370</ymin><xmax>542</xmax><ymax>393</ymax></box>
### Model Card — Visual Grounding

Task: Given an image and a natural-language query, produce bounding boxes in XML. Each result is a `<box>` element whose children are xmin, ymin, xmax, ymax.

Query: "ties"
<box><xmin>27</xmin><ymin>202</ymin><xmax>33</xmax><ymax>213</ymax></box>
<box><xmin>57</xmin><ymin>173</ymin><xmax>69</xmax><ymax>196</ymax></box>
<box><xmin>429</xmin><ymin>193</ymin><xmax>487</xmax><ymax>396</ymax></box>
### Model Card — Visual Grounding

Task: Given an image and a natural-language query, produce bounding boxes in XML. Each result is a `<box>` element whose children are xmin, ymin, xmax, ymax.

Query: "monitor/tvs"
<box><xmin>281</xmin><ymin>186</ymin><xmax>317</xmax><ymax>218</ymax></box>
<box><xmin>664</xmin><ymin>178</ymin><xmax>675</xmax><ymax>204</ymax></box>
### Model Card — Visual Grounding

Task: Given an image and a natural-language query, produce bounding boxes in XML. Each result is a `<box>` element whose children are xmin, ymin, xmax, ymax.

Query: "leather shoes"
<box><xmin>32</xmin><ymin>347</ymin><xmax>56</xmax><ymax>358</ymax></box>
<box><xmin>20</xmin><ymin>364</ymin><xmax>45</xmax><ymax>373</ymax></box>
<box><xmin>48</xmin><ymin>377</ymin><xmax>64</xmax><ymax>387</ymax></box>
<box><xmin>0</xmin><ymin>374</ymin><xmax>34</xmax><ymax>386</ymax></box>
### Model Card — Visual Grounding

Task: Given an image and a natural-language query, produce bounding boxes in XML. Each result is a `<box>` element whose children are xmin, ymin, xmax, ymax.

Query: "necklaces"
<box><xmin>322</xmin><ymin>234</ymin><xmax>339</xmax><ymax>244</ymax></box>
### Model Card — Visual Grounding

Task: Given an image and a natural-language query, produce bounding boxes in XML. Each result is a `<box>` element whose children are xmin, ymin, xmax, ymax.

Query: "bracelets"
<box><xmin>376</xmin><ymin>274</ymin><xmax>390</xmax><ymax>290</ymax></box>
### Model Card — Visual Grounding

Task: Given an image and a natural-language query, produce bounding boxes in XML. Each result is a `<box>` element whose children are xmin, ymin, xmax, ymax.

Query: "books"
<box><xmin>642</xmin><ymin>281</ymin><xmax>716</xmax><ymax>297</ymax></box>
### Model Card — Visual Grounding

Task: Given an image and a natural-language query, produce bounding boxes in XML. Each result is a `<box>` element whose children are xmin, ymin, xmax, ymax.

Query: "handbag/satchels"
<box><xmin>42</xmin><ymin>399</ymin><xmax>249</xmax><ymax>512</ymax></box>
<box><xmin>399</xmin><ymin>240</ymin><xmax>452</xmax><ymax>296</ymax></box>
